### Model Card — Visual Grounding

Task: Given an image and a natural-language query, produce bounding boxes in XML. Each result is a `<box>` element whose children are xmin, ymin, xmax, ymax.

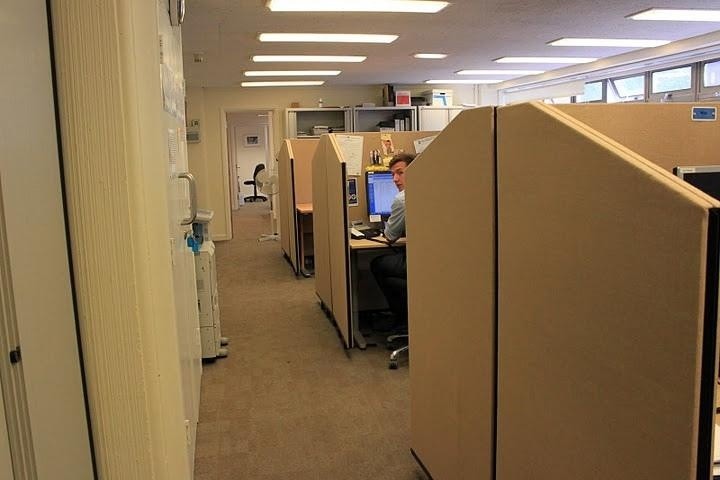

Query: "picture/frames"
<box><xmin>243</xmin><ymin>133</ymin><xmax>261</xmax><ymax>147</ymax></box>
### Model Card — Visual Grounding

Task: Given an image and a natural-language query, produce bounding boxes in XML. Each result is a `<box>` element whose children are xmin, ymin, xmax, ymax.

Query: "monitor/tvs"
<box><xmin>365</xmin><ymin>170</ymin><xmax>400</xmax><ymax>222</ymax></box>
<box><xmin>675</xmin><ymin>165</ymin><xmax>720</xmax><ymax>202</ymax></box>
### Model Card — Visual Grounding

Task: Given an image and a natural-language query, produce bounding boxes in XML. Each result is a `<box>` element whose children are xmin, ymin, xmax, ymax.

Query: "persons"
<box><xmin>370</xmin><ymin>154</ymin><xmax>416</xmax><ymax>334</ymax></box>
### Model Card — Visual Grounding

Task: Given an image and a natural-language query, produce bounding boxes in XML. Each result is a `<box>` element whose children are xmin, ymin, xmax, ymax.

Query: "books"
<box><xmin>311</xmin><ymin>125</ymin><xmax>345</xmax><ymax>136</ymax></box>
<box><xmin>379</xmin><ymin>117</ymin><xmax>410</xmax><ymax>131</ymax></box>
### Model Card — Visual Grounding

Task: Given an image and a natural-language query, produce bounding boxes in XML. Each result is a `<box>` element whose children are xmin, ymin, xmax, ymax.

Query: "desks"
<box><xmin>267</xmin><ymin>137</ymin><xmax>321</xmax><ymax>281</ymax></box>
<box><xmin>311</xmin><ymin>131</ymin><xmax>444</xmax><ymax>369</ymax></box>
<box><xmin>403</xmin><ymin>100</ymin><xmax>719</xmax><ymax>478</ymax></box>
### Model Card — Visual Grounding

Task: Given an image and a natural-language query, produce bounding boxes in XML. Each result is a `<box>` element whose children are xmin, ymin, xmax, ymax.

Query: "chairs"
<box><xmin>243</xmin><ymin>162</ymin><xmax>269</xmax><ymax>204</ymax></box>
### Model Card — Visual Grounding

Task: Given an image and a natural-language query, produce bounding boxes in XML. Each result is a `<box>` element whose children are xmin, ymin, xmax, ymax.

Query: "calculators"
<box><xmin>352</xmin><ymin>220</ymin><xmax>371</xmax><ymax>230</ymax></box>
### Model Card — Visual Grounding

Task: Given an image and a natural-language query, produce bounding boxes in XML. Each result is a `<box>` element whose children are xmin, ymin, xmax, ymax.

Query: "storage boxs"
<box><xmin>422</xmin><ymin>89</ymin><xmax>454</xmax><ymax>107</ymax></box>
<box><xmin>395</xmin><ymin>90</ymin><xmax>412</xmax><ymax>107</ymax></box>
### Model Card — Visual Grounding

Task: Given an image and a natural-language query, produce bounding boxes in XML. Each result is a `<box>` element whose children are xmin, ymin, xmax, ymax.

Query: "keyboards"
<box><xmin>377</xmin><ymin>227</ymin><xmax>406</xmax><ymax>238</ymax></box>
